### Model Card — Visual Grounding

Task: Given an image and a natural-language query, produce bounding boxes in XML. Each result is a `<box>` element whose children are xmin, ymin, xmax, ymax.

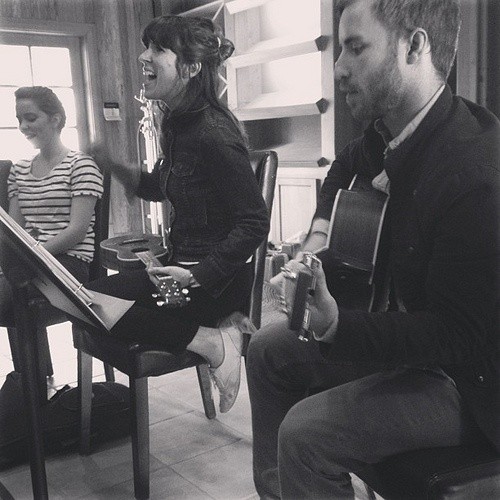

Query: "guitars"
<box><xmin>281</xmin><ymin>174</ymin><xmax>392</xmax><ymax>344</ymax></box>
<box><xmin>100</xmin><ymin>233</ymin><xmax>193</xmax><ymax>309</ymax></box>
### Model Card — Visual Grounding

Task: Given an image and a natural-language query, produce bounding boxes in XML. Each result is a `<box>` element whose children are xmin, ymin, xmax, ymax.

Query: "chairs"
<box><xmin>72</xmin><ymin>151</ymin><xmax>279</xmax><ymax>500</ymax></box>
<box><xmin>18</xmin><ymin>158</ymin><xmax>116</xmax><ymax>384</ymax></box>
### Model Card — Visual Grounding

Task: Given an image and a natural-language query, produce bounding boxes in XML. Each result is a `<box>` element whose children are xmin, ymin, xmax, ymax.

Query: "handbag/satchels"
<box><xmin>0</xmin><ymin>372</ymin><xmax>33</xmax><ymax>472</ymax></box>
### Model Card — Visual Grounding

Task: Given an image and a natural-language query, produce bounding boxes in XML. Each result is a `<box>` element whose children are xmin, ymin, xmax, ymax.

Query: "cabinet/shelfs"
<box><xmin>174</xmin><ymin>0</ymin><xmax>357</xmax><ymax>246</ymax></box>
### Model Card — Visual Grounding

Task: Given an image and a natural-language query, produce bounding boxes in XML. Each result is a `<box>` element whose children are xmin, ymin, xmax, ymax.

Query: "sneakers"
<box><xmin>208</xmin><ymin>327</ymin><xmax>243</xmax><ymax>413</ymax></box>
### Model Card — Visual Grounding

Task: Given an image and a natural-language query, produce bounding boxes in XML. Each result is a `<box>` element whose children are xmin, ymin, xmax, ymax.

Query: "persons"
<box><xmin>83</xmin><ymin>14</ymin><xmax>270</xmax><ymax>413</ymax></box>
<box><xmin>247</xmin><ymin>1</ymin><xmax>500</xmax><ymax>500</ymax></box>
<box><xmin>0</xmin><ymin>86</ymin><xmax>104</xmax><ymax>327</ymax></box>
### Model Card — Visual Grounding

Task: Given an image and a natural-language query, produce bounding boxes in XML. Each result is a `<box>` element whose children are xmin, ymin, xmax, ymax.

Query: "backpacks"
<box><xmin>51</xmin><ymin>382</ymin><xmax>132</xmax><ymax>443</ymax></box>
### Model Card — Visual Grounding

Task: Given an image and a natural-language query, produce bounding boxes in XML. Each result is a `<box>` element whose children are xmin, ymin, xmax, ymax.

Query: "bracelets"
<box><xmin>189</xmin><ymin>273</ymin><xmax>196</xmax><ymax>286</ymax></box>
